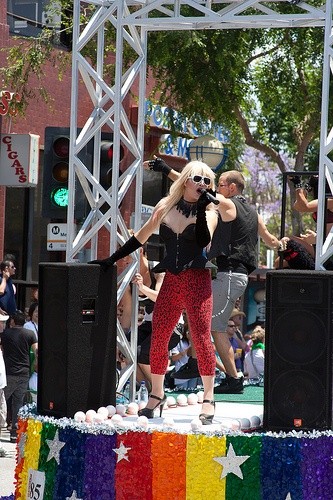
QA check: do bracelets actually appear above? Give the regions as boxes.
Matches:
[295,188,301,192]
[180,352,183,356]
[278,241,283,252]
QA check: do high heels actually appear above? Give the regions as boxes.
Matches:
[199,400,215,424]
[138,393,167,418]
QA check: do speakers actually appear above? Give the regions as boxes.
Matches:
[35,262,116,419]
[263,269,333,433]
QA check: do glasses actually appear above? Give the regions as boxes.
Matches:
[216,182,230,189]
[7,265,16,269]
[188,175,211,186]
[227,324,237,328]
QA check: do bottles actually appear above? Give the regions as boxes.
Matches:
[123,381,130,404]
[138,381,148,403]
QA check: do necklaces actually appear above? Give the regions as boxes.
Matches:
[176,194,207,219]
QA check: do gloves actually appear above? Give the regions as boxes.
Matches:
[87,234,142,271]
[193,188,217,247]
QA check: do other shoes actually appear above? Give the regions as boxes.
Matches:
[10,433,19,443]
[214,372,245,394]
[0,447,7,457]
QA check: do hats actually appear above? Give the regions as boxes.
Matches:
[229,307,247,319]
[0,313,9,321]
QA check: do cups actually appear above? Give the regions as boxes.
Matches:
[236,348,242,357]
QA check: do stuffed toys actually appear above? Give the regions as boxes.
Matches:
[274,239,315,270]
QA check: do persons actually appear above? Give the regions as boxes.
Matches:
[161,297,265,393]
[0,254,39,457]
[119,228,186,399]
[142,154,282,394]
[293,174,333,271]
[88,160,233,422]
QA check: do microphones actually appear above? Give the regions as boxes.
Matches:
[201,189,219,205]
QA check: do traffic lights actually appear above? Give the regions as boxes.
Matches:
[88,131,124,219]
[42,127,88,220]
